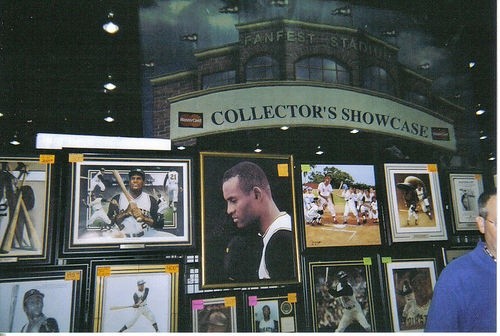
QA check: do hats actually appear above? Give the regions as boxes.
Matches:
[22,288,44,303]
[205,313,228,326]
[226,233,265,282]
[137,279,145,285]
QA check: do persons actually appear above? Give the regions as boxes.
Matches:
[0,159,478,333]
[424,187,497,333]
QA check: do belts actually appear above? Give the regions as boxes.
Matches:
[125,231,144,238]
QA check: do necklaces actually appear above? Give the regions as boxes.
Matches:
[262,212,287,237]
[484,245,497,263]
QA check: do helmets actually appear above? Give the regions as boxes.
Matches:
[338,271,348,279]
[128,168,145,180]
[395,175,427,196]
